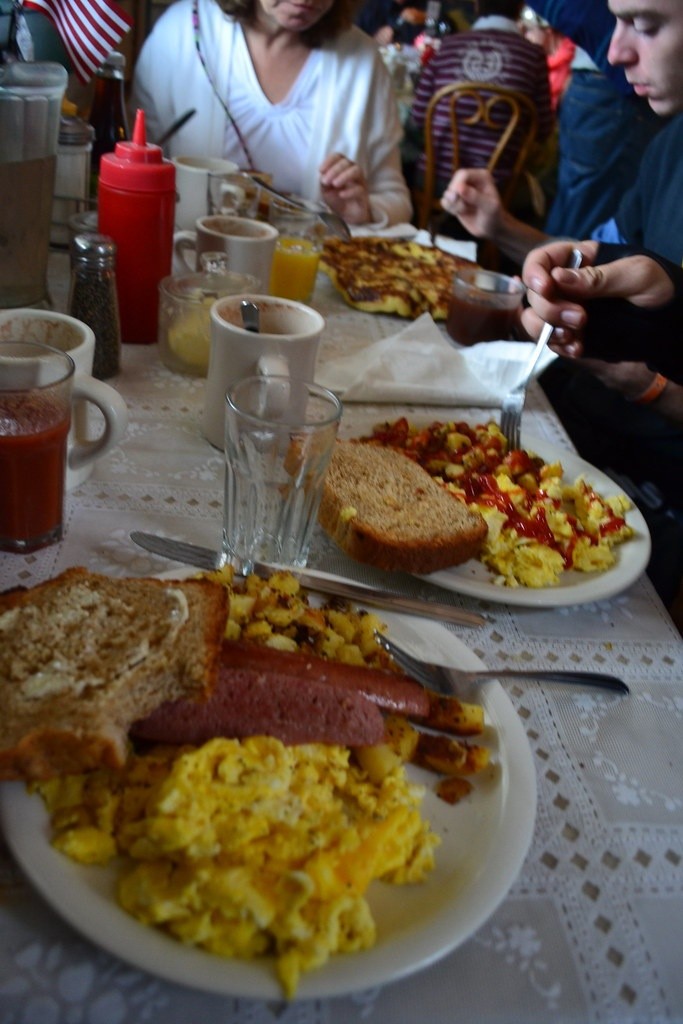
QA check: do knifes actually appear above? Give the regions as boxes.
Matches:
[131,531,486,629]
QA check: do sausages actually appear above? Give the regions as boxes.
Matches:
[116,639,433,747]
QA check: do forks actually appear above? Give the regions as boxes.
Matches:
[373,629,630,696]
[500,250,584,454]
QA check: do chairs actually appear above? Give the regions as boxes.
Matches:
[406,83,539,246]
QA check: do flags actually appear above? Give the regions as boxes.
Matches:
[20,0,136,86]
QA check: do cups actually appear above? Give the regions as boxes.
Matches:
[220,375,343,572]
[268,194,331,307]
[449,269,525,346]
[203,295,325,454]
[172,155,245,228]
[0,307,128,491]
[159,271,259,379]
[1,338,73,556]
[172,215,279,296]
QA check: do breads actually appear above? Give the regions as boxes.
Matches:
[0,565,231,785]
[282,440,487,577]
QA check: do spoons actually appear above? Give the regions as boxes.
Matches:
[242,172,353,243]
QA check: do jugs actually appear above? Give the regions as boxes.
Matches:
[0,62,69,321]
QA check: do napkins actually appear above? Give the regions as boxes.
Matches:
[308,311,559,404]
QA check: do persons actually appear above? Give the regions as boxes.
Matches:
[129,0,417,234]
[348,0,683,606]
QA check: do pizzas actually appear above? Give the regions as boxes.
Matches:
[311,235,489,320]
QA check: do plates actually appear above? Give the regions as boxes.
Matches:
[407,448,651,608]
[1,565,540,1002]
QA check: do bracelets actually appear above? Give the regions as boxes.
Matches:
[625,371,668,407]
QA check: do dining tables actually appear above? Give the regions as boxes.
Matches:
[0,268,683,1024]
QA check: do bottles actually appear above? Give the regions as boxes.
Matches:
[97,108,175,345]
[47,121,93,246]
[67,230,122,382]
